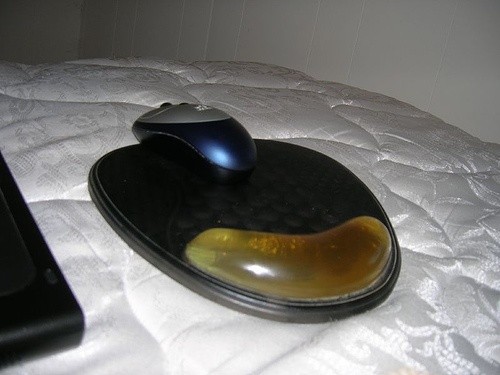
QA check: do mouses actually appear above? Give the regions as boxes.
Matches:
[131,102,258,180]
